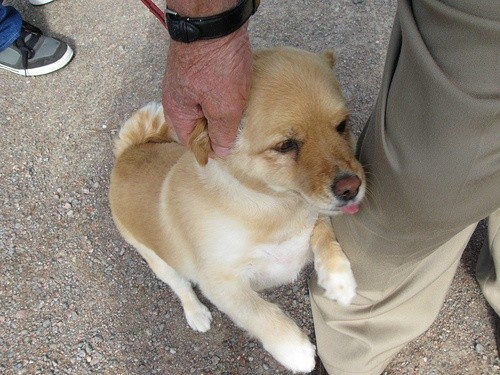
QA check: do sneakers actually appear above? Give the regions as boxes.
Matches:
[0,23,73,75]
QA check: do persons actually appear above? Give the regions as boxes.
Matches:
[0,0,73,77]
[162,0,499,375]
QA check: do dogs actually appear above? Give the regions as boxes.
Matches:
[108,44,369,375]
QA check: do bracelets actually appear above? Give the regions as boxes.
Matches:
[142,0,261,42]
